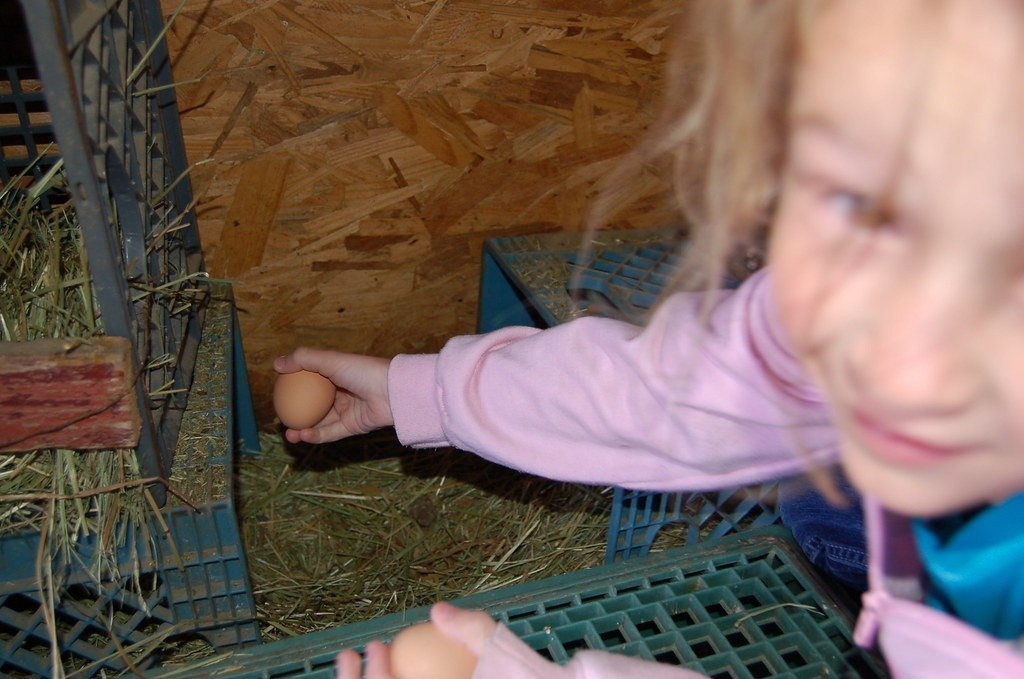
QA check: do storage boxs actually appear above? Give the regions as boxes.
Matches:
[0,0,214,517]
[121,526,892,678]
[473,227,789,565]
[0,281,263,679]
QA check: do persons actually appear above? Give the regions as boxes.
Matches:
[273,0,1024,679]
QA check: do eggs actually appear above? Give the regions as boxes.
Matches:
[389,622,476,679]
[273,370,336,430]
